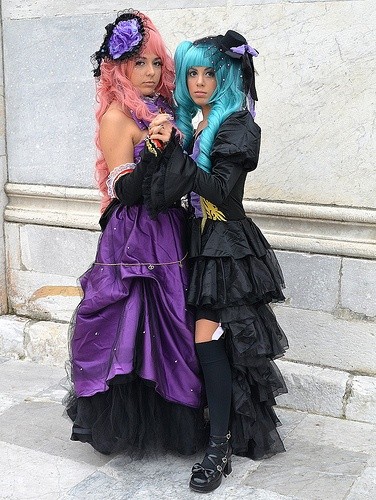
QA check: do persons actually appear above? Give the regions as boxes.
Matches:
[148,31,283,493]
[67,7,210,459]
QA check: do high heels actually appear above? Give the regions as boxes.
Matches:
[187,439,234,493]
